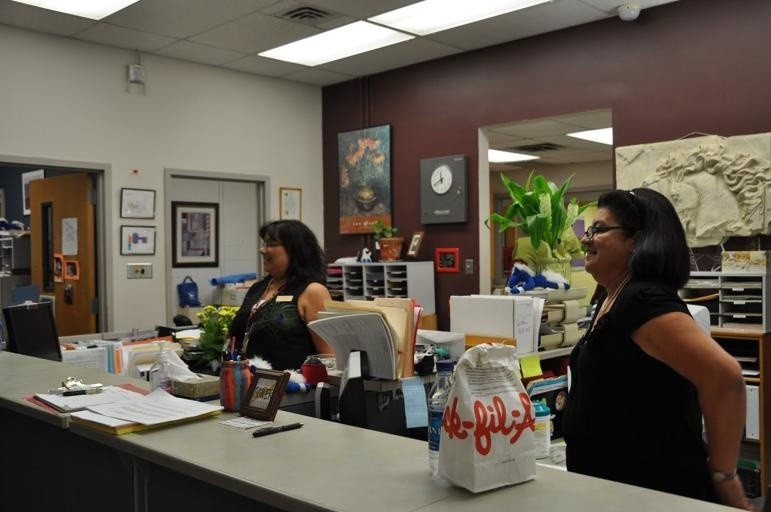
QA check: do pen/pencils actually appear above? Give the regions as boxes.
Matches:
[253,423,303,437]
[63,389,102,395]
[223,336,247,361]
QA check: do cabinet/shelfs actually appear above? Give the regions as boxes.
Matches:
[325,261,437,331]
[680,262,771,333]
[709,326,770,512]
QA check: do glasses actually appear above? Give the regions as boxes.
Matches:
[585,224,631,239]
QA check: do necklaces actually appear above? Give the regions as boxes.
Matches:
[599,272,630,311]
[255,278,286,315]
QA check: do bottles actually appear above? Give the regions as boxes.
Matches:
[427,360,457,479]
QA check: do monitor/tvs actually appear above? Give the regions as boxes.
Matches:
[3,301,62,361]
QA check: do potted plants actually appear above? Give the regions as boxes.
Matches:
[484,170,597,286]
[372,220,404,262]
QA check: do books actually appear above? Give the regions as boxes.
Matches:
[307,288,593,398]
[32,382,219,436]
[682,277,762,377]
[60,327,205,379]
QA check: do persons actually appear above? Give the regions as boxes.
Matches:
[232,219,333,370]
[561,188,756,511]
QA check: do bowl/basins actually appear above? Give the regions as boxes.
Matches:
[309,354,337,370]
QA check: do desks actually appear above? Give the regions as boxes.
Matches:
[0,349,216,512]
[73,395,749,511]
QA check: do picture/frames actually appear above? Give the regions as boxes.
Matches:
[336,125,393,234]
[120,187,156,219]
[407,231,425,257]
[120,225,156,256]
[433,248,460,273]
[170,201,219,268]
[280,188,302,223]
[127,263,153,280]
[238,369,291,423]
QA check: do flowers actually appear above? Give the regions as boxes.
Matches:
[194,303,242,365]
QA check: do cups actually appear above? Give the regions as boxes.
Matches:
[219,360,253,410]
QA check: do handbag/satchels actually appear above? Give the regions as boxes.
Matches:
[433,333,539,497]
[178,276,201,307]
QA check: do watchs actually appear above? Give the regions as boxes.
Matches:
[709,469,735,482]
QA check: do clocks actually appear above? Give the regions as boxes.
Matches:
[419,155,467,224]
